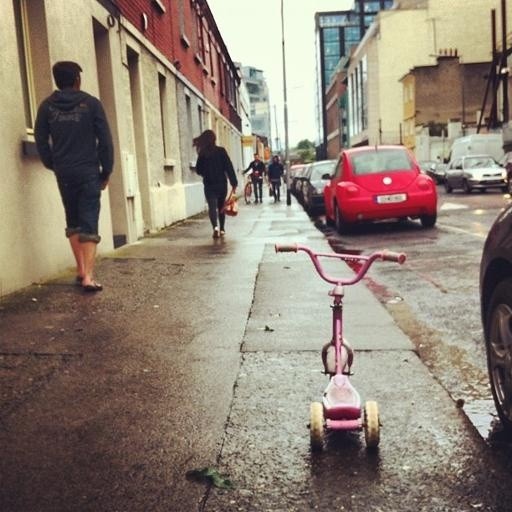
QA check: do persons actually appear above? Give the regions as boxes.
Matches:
[268,155,286,204]
[189,130,237,236]
[240,153,268,203]
[33,62,115,291]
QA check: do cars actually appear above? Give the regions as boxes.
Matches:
[426,164,448,184]
[480,202,512,432]
[290,145,437,235]
[443,155,509,194]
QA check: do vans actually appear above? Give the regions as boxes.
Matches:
[446,135,510,167]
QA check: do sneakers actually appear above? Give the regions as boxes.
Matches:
[75,275,104,292]
[211,226,225,238]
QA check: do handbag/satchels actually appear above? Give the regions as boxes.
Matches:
[219,188,240,217]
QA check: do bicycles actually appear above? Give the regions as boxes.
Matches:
[242,172,258,205]
